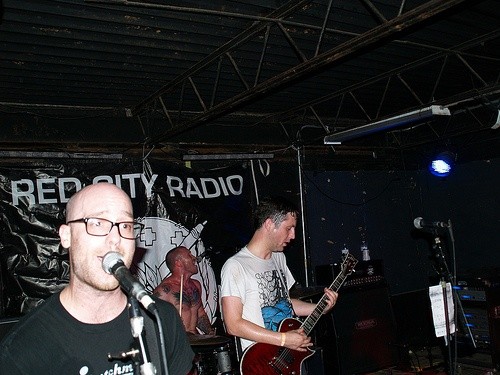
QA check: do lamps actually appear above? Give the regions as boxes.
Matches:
[431,147,458,174]
[324,105,451,145]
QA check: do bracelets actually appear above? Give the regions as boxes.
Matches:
[281,332,286,347]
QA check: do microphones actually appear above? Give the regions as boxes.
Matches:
[102,251,157,314]
[414,217,445,229]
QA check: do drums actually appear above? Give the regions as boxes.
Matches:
[188,336,239,375]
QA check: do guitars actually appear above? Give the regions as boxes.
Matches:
[240,254,359,375]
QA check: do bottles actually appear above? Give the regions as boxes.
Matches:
[360,240,368,255]
[341,244,350,254]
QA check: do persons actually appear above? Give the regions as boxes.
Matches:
[220,198,338,352]
[0,181,196,375]
[153,246,214,336]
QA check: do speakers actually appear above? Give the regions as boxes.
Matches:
[295,277,401,375]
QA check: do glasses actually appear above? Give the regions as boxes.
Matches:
[67,218,145,240]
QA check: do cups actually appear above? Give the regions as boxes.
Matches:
[363,250,371,260]
